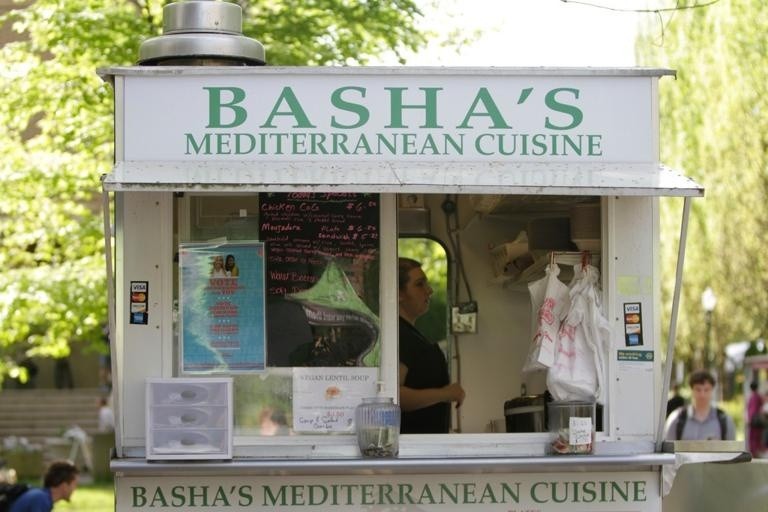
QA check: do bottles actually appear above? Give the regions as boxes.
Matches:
[354,396,403,460]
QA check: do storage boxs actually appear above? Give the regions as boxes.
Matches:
[145,379,234,459]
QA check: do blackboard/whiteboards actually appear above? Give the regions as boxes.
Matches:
[258,192,381,368]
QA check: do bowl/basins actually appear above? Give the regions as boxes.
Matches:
[571,237,602,252]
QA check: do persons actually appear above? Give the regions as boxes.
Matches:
[666,383,685,415]
[95,395,115,433]
[745,380,768,458]
[208,254,227,278]
[224,254,240,277]
[0,461,79,512]
[398,256,465,433]
[662,367,738,440]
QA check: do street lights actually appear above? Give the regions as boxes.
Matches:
[701,286,716,369]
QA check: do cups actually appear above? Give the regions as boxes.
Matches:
[550,400,598,455]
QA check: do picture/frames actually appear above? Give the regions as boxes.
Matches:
[178,240,268,375]
